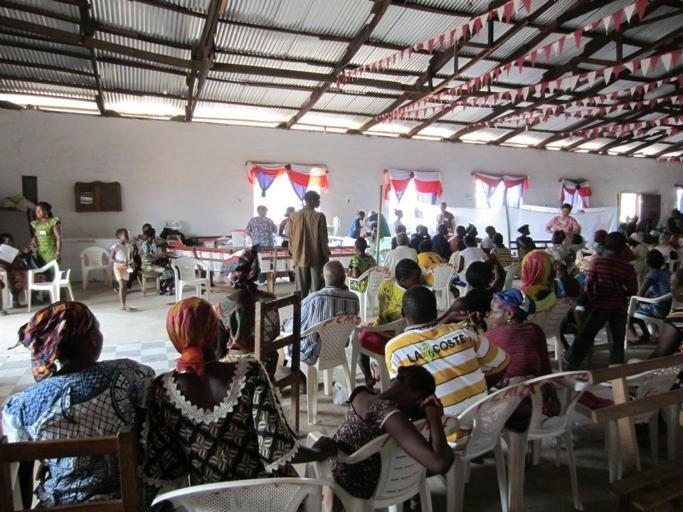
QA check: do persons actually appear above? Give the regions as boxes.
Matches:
[2,190,682,511]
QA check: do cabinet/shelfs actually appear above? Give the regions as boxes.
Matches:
[0,207,34,248]
[640,193,660,223]
[74,180,123,212]
